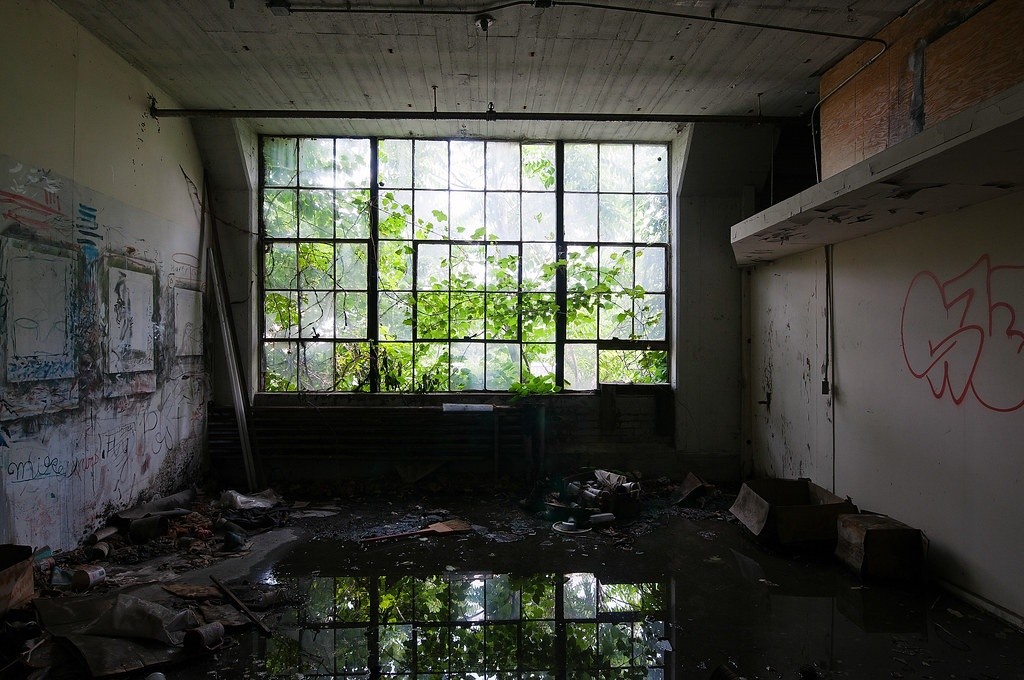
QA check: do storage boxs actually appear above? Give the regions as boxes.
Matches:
[835,513,925,578]
[0,544,37,614]
[732,477,851,543]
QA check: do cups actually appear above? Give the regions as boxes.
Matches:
[73,566,105,593]
[186,622,225,651]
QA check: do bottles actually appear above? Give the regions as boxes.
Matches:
[560,479,615,530]
[220,518,249,552]
